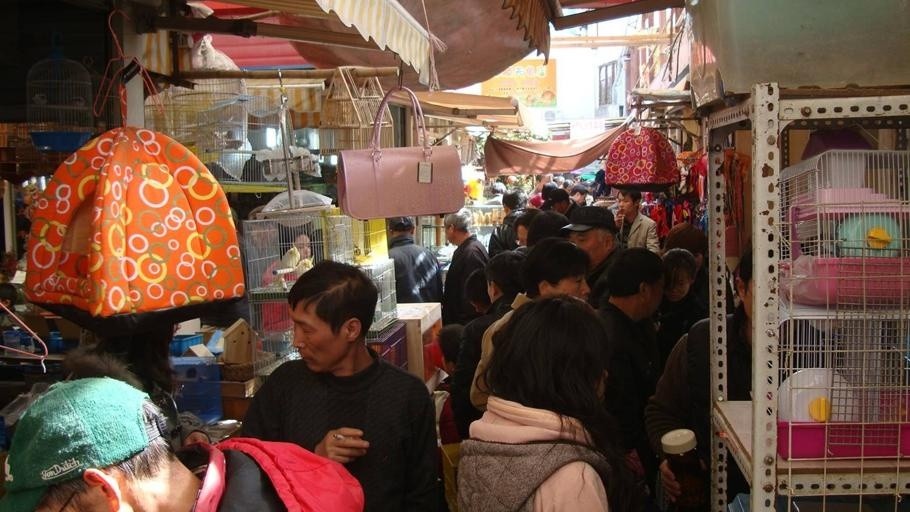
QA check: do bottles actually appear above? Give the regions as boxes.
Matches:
[660,426,707,509]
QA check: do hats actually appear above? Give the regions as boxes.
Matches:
[546,189,569,206]
[560,206,614,234]
[0,375,168,512]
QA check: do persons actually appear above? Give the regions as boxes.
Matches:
[387,215,443,304]
[260,232,316,333]
[1,376,366,512]
[236,259,442,512]
[76,359,212,447]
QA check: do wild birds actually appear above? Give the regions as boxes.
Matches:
[280,246,301,268]
[296,259,313,279]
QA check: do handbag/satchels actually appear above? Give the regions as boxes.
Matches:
[331,88,465,218]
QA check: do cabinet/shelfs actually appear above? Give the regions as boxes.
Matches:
[701,72,907,510]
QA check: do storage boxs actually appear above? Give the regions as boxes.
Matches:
[397,301,446,393]
[365,319,409,373]
